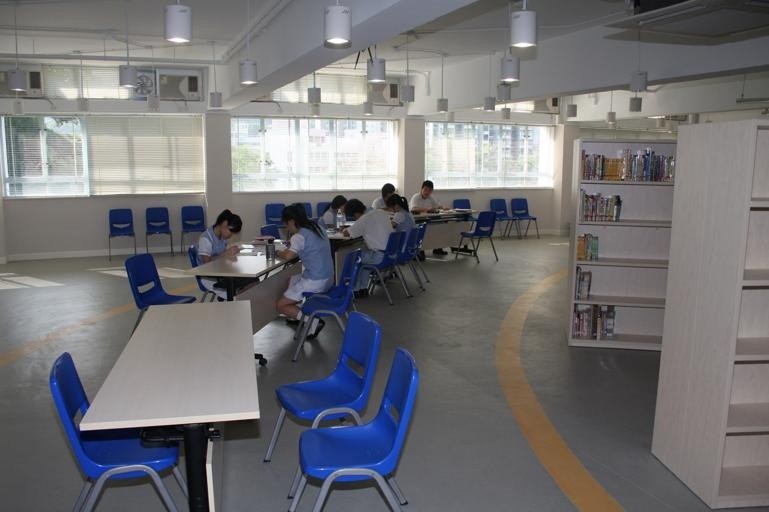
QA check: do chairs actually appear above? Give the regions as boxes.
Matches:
[354,231,412,306]
[453,199,478,231]
[146,207,174,256]
[260,224,280,240]
[264,310,381,498]
[289,345,419,511]
[301,247,362,319]
[490,198,519,240]
[370,227,426,295]
[49,352,188,512]
[180,206,205,256]
[108,208,137,261]
[188,244,240,303]
[265,203,290,239]
[317,202,331,219]
[455,211,498,264]
[510,198,540,239]
[304,202,312,221]
[125,253,197,339]
[388,222,430,291]
[293,256,363,362]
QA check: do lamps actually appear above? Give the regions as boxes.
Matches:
[436,57,448,112]
[164,0,191,43]
[147,48,161,112]
[566,95,576,117]
[401,35,414,102]
[608,91,616,125]
[239,1,258,85]
[367,44,385,82]
[119,23,138,88]
[501,49,520,82]
[210,43,222,106]
[632,29,648,92]
[309,102,320,117]
[363,100,373,115]
[510,0,536,49]
[323,0,352,49]
[496,82,511,101]
[77,58,89,112]
[502,104,510,119]
[12,92,23,115]
[484,55,495,111]
[308,72,321,103]
[630,92,643,111]
[6,7,27,92]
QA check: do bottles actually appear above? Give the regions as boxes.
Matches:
[265,237,277,260]
[336,210,344,230]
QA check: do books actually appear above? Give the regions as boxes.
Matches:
[421,207,473,216]
[571,304,616,339]
[326,224,352,234]
[577,234,599,263]
[576,267,593,300]
[229,236,289,256]
[582,190,623,222]
[582,147,674,182]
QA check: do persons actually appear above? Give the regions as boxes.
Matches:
[196,210,242,302]
[371,182,396,210]
[343,198,390,300]
[275,201,335,341]
[386,194,416,279]
[409,180,451,262]
[321,196,348,228]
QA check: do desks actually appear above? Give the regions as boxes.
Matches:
[79,300,260,512]
[414,209,481,257]
[188,238,299,367]
[325,221,364,290]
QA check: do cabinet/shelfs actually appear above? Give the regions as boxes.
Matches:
[651,119,768,508]
[567,137,678,351]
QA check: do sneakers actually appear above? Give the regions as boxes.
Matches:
[286,317,300,326]
[354,290,361,299]
[419,251,425,262]
[307,318,325,340]
[433,248,448,255]
[362,289,369,297]
